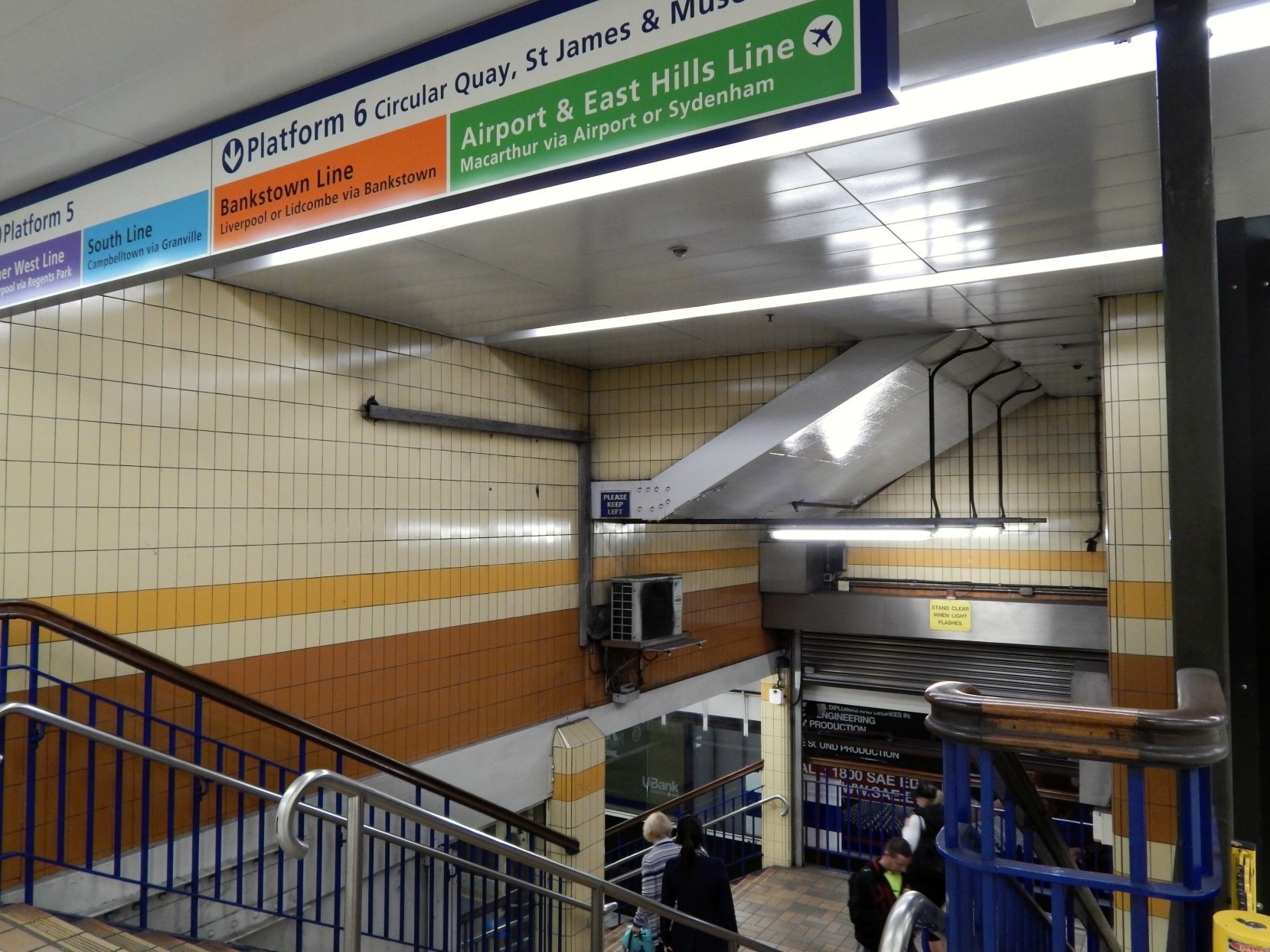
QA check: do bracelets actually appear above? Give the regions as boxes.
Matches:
[633,922,642,928]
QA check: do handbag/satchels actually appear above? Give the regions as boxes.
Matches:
[622,925,655,952]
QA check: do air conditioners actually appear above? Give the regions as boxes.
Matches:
[610,572,684,643]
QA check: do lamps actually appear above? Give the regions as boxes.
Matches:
[767,521,1006,541]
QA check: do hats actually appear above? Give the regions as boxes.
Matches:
[910,783,936,799]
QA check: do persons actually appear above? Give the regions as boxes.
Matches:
[632,812,741,952]
[849,784,946,952]
